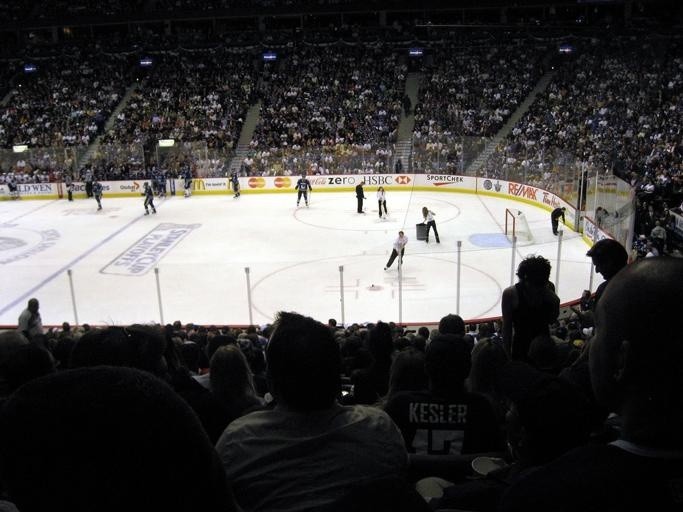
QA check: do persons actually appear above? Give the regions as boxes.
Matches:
[500,256,561,363]
[527,0,683,512]
[1,298,529,512]
[1,1,527,215]
[384,231,408,271]
[421,207,441,245]
